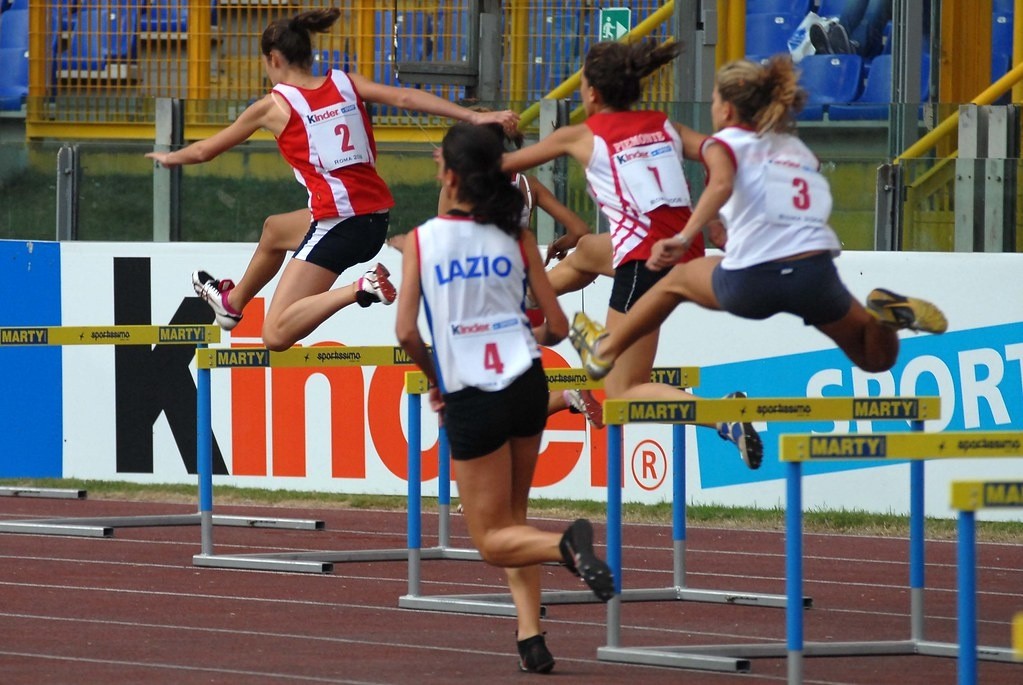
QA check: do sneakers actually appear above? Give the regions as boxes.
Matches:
[569,311,615,381]
[516,630,555,673]
[866,287,948,335]
[192,269,244,330]
[568,390,606,429]
[356,263,397,308]
[560,519,615,600]
[717,392,764,470]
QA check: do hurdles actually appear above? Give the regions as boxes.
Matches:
[594,395,1023,674]
[0,324,326,539]
[776,430,1023,685]
[395,364,813,619]
[947,479,1023,685]
[190,345,565,574]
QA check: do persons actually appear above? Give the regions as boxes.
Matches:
[395,121,615,673]
[386,106,604,430]
[433,41,763,469]
[568,55,948,374]
[144,7,522,352]
[809,0,932,58]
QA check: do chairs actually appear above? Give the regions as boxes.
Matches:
[245,0,675,125]
[0,0,224,118]
[745,0,1013,120]
